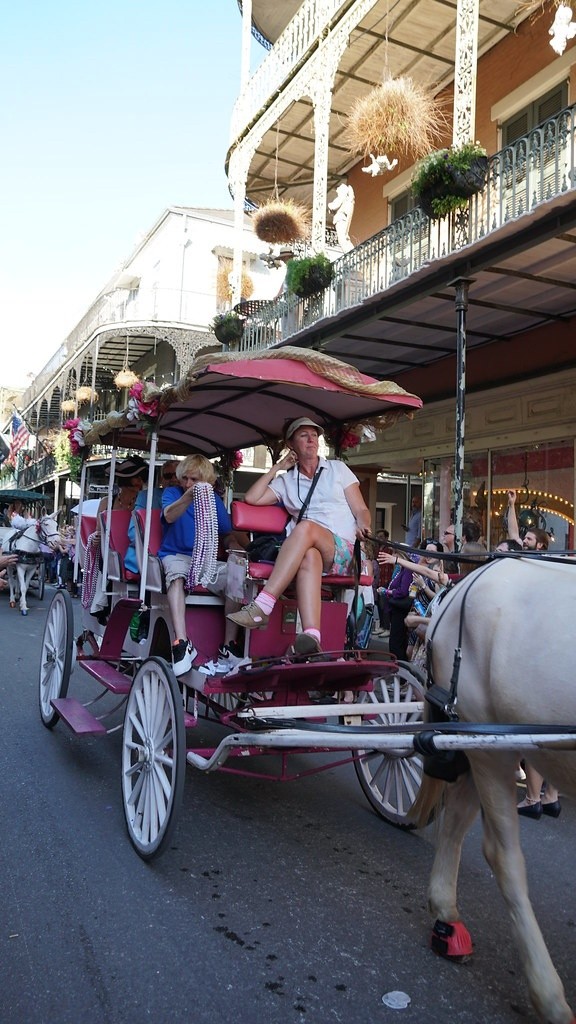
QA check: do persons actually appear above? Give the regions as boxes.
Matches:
[91,452,150,612]
[1,503,81,598]
[225,417,372,660]
[123,460,183,643]
[43,439,54,457]
[274,246,299,340]
[158,455,252,676]
[328,183,360,271]
[361,491,560,818]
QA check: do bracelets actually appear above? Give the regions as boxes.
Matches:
[395,556,398,564]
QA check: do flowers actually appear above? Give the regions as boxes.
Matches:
[325,428,360,459]
[228,449,245,470]
[124,381,159,438]
[207,313,245,344]
[63,419,94,453]
[412,140,489,219]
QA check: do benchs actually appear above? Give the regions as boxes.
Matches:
[87,496,375,657]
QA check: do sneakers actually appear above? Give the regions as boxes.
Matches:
[217,640,247,667]
[171,636,198,678]
[225,600,269,631]
[292,631,324,661]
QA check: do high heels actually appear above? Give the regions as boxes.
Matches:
[539,800,562,818]
[514,801,543,820]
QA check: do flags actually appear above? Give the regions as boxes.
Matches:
[0,409,30,463]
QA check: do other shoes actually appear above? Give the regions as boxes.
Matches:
[514,777,529,788]
[51,580,66,589]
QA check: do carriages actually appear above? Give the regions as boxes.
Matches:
[38,344,575,1023]
[0,490,62,613]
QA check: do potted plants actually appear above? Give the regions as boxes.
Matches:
[285,254,332,297]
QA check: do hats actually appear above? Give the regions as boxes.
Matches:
[101,454,150,483]
[284,416,325,445]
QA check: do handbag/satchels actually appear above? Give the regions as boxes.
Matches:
[247,535,282,564]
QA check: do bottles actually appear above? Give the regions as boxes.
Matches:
[413,599,426,616]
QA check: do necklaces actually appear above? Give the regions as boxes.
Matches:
[186,482,218,588]
[81,532,100,609]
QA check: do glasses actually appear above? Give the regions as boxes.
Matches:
[162,472,178,481]
[425,539,438,545]
[443,530,455,536]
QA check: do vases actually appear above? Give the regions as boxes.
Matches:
[421,156,486,218]
[213,319,243,342]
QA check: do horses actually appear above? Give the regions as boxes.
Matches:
[406,555,576,1024]
[1,506,61,610]
